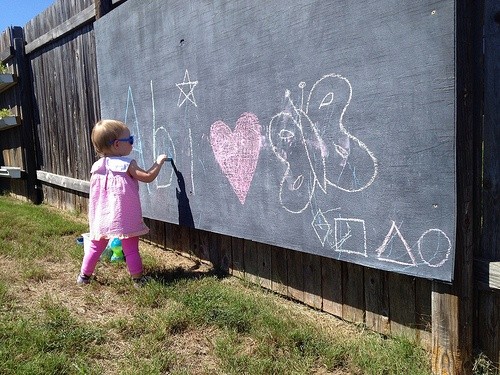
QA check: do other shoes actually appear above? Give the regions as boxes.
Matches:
[132,275,153,288]
[77,272,96,283]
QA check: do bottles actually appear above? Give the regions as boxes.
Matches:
[110,238,124,263]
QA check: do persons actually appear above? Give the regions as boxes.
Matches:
[77,119,168,286]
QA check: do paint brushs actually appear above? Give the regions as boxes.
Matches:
[161,157,172,161]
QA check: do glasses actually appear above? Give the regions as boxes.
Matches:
[108,135,134,146]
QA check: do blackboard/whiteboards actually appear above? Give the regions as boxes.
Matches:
[92,0,457,282]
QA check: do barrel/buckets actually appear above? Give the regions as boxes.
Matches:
[81,232,107,261]
